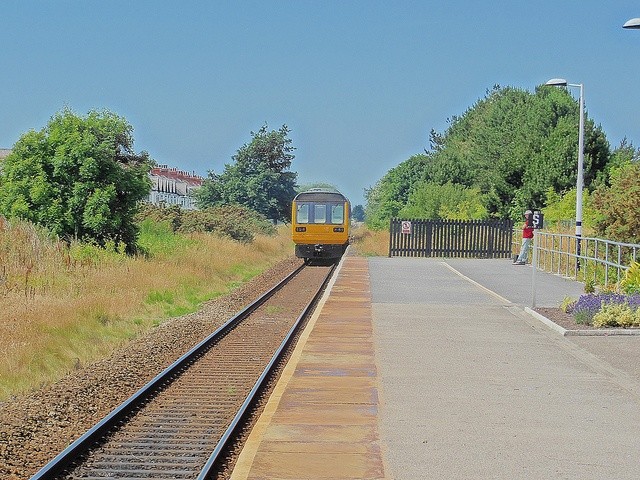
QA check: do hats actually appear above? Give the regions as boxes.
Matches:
[524,210,532,214]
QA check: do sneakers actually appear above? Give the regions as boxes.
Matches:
[521,262,525,265]
[512,261,521,265]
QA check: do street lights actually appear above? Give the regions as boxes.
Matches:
[624,18,640,28]
[545,79,584,270]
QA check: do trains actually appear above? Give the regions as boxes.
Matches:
[292,187,351,263]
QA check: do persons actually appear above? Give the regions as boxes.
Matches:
[512,210,534,265]
